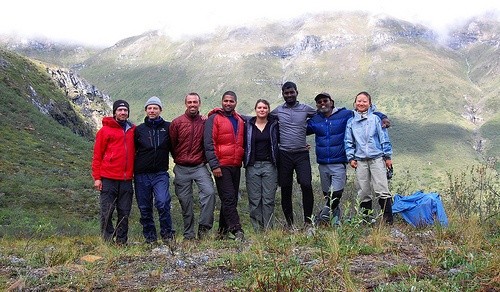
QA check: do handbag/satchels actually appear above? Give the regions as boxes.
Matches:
[392,191,448,228]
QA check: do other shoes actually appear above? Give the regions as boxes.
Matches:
[234,231,245,241]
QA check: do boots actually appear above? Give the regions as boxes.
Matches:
[359,199,372,226]
[378,197,394,227]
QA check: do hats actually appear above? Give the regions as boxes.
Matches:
[314,92,331,101]
[113,99,130,117]
[144,96,162,111]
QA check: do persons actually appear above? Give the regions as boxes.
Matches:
[306,93,391,224]
[92,100,137,245]
[134,96,176,246]
[204,91,245,240]
[344,91,394,225]
[238,82,316,231]
[244,99,311,231]
[168,93,216,242]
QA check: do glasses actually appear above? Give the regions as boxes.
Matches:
[317,99,328,104]
